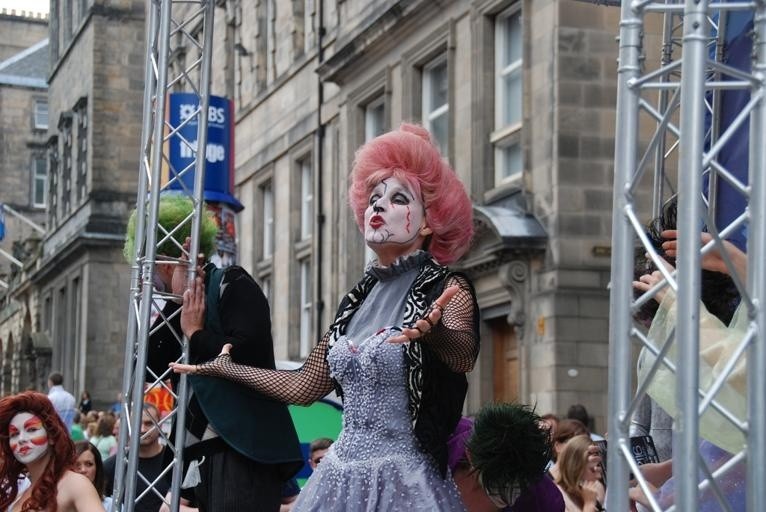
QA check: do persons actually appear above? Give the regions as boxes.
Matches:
[1,370,166,512]
[628,391,675,509]
[631,227,750,460]
[165,121,482,512]
[449,402,567,510]
[628,196,742,344]
[280,438,335,512]
[538,405,608,510]
[123,193,305,512]
